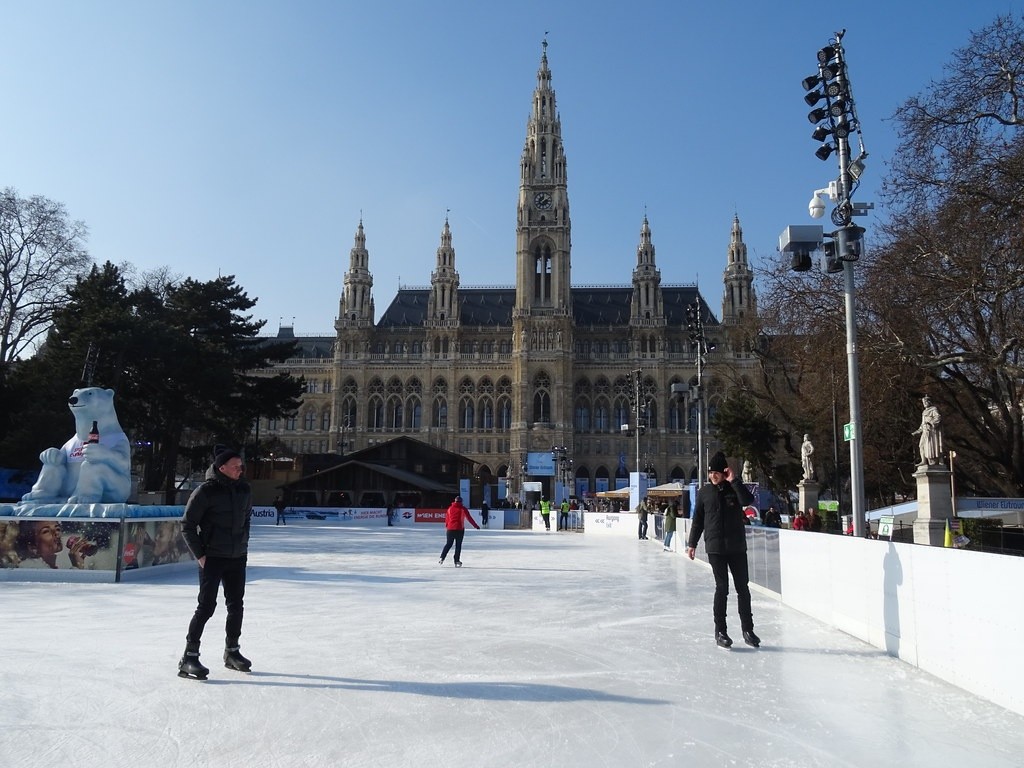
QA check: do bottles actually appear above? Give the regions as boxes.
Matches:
[89,421,99,445]
[54,534,98,556]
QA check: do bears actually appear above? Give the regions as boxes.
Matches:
[20,385,132,504]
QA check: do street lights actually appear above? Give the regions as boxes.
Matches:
[671,294,709,491]
[620,367,646,472]
[551,445,571,486]
[779,26,875,539]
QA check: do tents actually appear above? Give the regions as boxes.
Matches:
[647,480,688,496]
[597,486,630,498]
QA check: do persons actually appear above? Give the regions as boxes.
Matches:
[539,496,551,528]
[437,495,480,568]
[793,510,811,531]
[741,460,752,482]
[0,521,88,569]
[688,450,761,648]
[663,500,680,552]
[481,500,492,529]
[180,444,252,680]
[911,396,941,467]
[124,522,180,571]
[847,517,853,536]
[386,504,394,526]
[273,495,287,525]
[638,497,664,540]
[764,507,783,529]
[559,496,686,530]
[805,507,823,533]
[494,498,527,510]
[800,434,815,481]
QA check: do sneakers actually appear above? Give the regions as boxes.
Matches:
[715,630,733,650]
[177,648,209,680]
[743,628,760,648]
[223,646,252,673]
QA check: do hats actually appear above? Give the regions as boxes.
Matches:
[455,496,463,502]
[214,444,242,470]
[708,452,728,475]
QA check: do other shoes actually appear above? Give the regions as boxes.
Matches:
[439,557,443,564]
[664,546,673,552]
[454,561,462,568]
[639,536,648,540]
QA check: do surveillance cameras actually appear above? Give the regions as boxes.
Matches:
[809,197,825,219]
